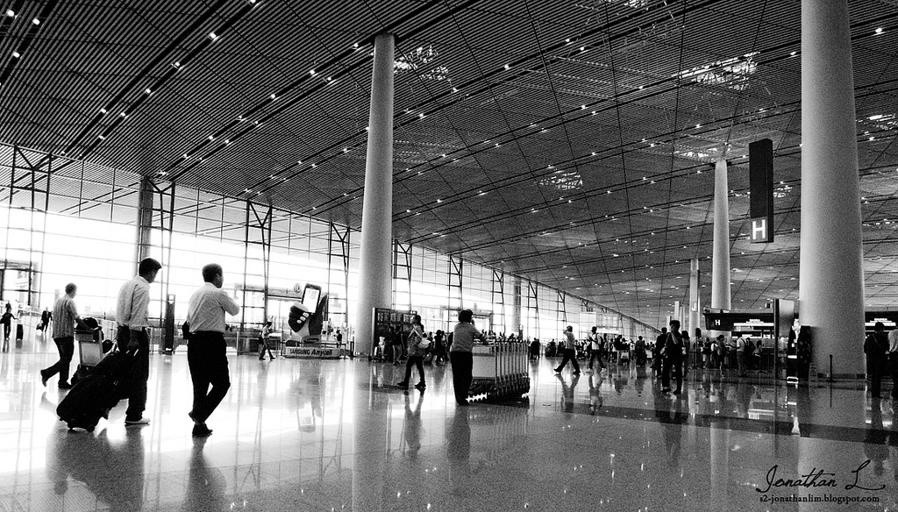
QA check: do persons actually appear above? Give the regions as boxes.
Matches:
[0,300,897,413]
[182,262,239,434]
[38,283,89,388]
[116,258,162,423]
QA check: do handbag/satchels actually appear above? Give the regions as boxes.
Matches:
[417,337,432,350]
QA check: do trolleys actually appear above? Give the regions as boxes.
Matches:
[465,338,529,400]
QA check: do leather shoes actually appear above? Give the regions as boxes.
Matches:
[59,383,73,389]
[415,381,426,387]
[189,409,213,436]
[397,381,409,387]
[125,417,151,423]
[41,369,51,387]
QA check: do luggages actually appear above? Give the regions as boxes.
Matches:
[57,345,143,432]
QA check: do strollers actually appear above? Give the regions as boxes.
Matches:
[71,326,120,387]
